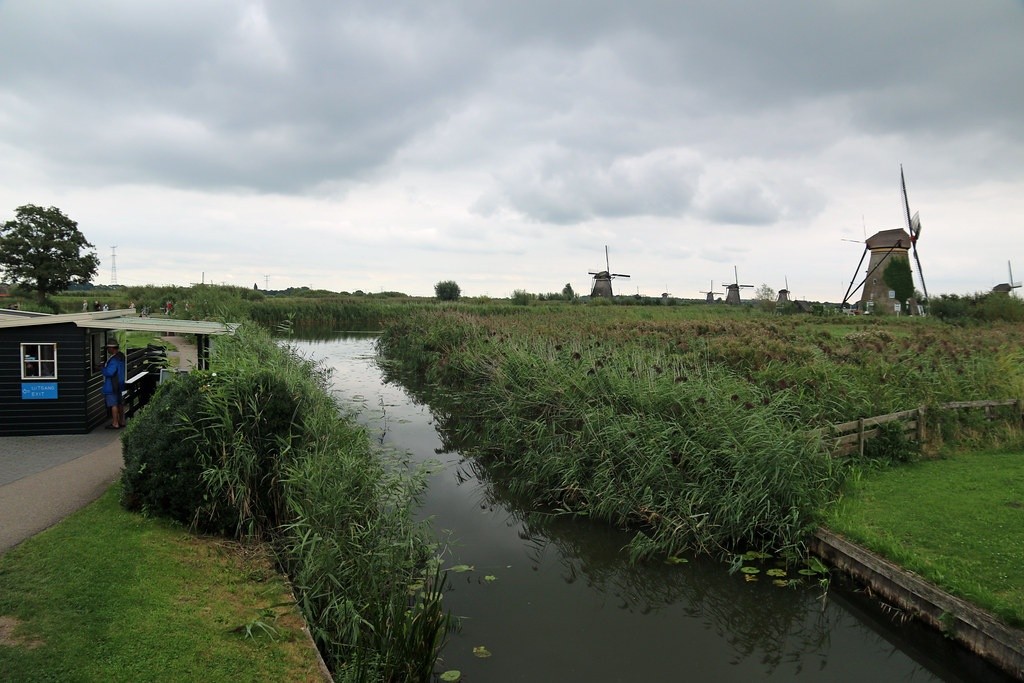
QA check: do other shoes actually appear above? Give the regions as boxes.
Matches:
[104,422,125,428]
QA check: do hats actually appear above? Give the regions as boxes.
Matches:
[107,337,118,347]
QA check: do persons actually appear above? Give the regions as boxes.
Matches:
[96,337,127,430]
[129,298,210,318]
[82,299,109,312]
